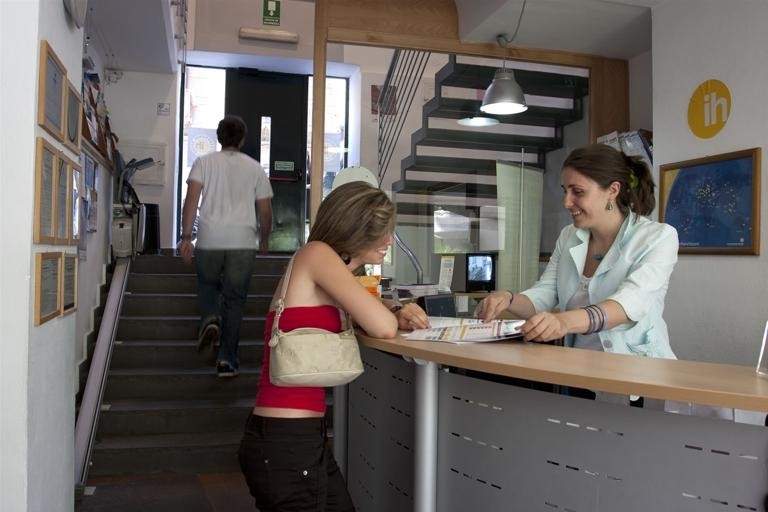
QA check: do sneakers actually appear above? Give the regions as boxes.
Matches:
[216,358,243,378]
[195,314,221,362]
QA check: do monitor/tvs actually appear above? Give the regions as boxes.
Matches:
[417,294,456,317]
[431,252,496,292]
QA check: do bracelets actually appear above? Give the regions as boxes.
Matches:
[179,233,192,241]
[579,303,608,336]
[388,305,402,314]
[503,289,516,305]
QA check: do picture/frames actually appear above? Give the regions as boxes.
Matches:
[656,146,765,259]
[32,37,86,328]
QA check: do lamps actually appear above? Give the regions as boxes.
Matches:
[478,0,532,117]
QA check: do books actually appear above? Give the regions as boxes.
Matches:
[400,314,526,345]
[390,281,439,298]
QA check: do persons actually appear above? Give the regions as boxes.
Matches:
[237,180,431,511]
[176,113,275,378]
[471,141,680,409]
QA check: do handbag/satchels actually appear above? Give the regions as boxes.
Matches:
[267,317,366,388]
